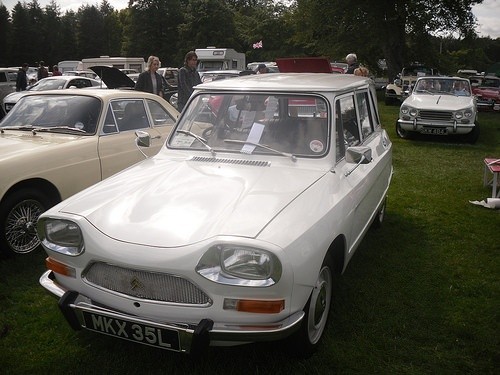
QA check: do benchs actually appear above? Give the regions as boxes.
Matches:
[256,117,364,147]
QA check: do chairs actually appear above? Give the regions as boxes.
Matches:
[109,103,149,132]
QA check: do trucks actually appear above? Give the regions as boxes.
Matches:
[194,46,246,75]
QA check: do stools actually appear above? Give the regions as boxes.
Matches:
[483,158,500,198]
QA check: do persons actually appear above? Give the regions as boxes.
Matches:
[16,61,62,94]
[135,56,165,97]
[345,54,359,75]
[450,81,471,96]
[177,51,202,113]
[258,64,275,73]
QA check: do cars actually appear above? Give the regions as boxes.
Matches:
[395,75,481,144]
[0,55,181,116]
[168,56,375,120]
[383,61,438,104]
[0,88,214,256]
[35,71,395,358]
[465,75,500,112]
